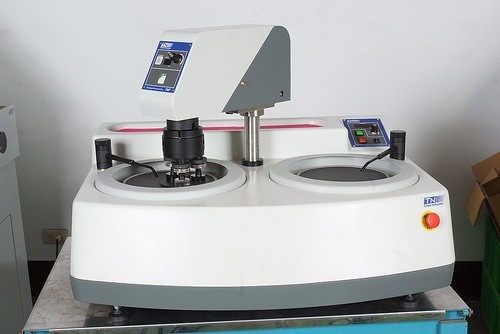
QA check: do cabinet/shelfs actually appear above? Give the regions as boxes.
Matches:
[1,104,33,334]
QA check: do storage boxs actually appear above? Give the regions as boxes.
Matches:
[465,151,500,239]
[480,262,500,334]
[483,213,500,301]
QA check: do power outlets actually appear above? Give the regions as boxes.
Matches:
[42,229,67,244]
